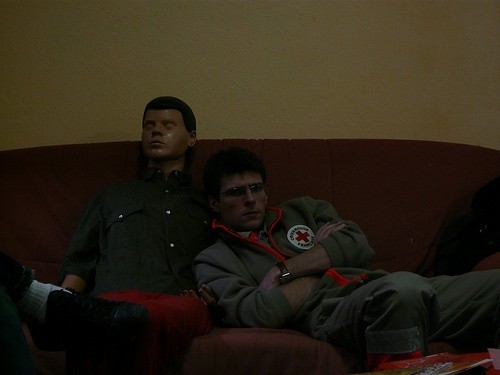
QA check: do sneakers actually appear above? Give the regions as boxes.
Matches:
[25,285,152,351]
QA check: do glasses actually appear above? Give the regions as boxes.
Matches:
[220,183,269,197]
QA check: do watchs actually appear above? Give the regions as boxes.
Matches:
[274,262,294,285]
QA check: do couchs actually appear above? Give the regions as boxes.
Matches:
[0,137,500,374]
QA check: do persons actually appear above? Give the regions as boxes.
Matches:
[64,96,220,310]
[191,143,500,375]
[0,253,144,374]
